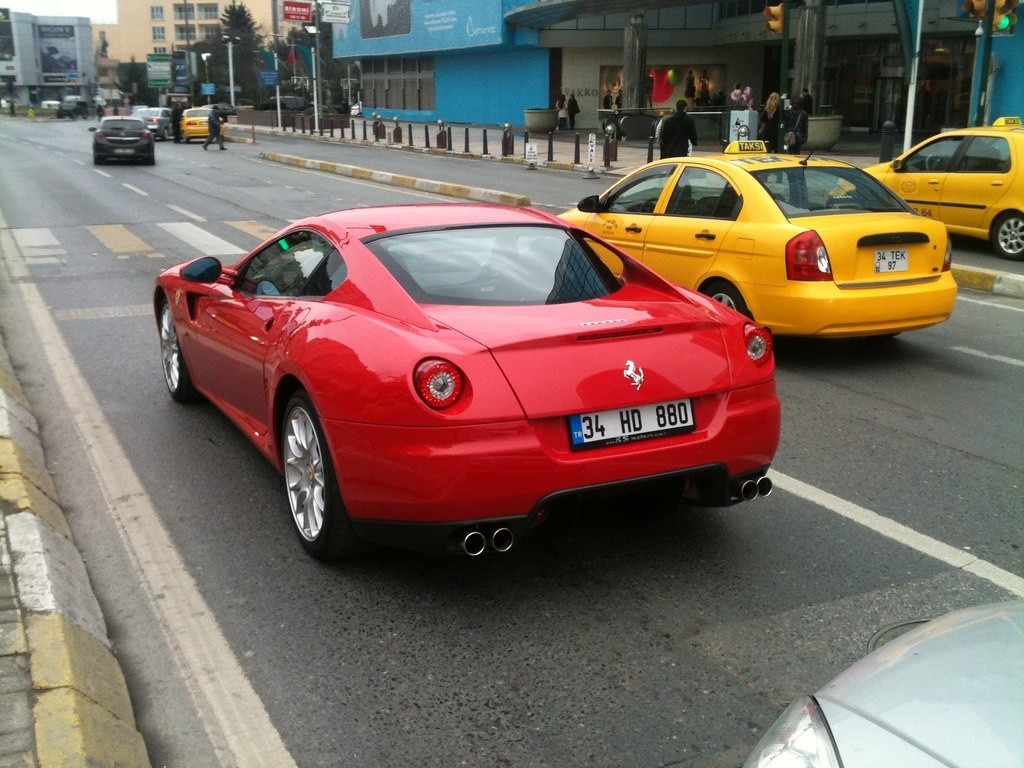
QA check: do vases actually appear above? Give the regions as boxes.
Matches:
[524,109,561,135]
[801,114,844,154]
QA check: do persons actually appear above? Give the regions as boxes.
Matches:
[96,105,104,122]
[112,106,119,115]
[171,103,182,143]
[183,102,191,109]
[8,102,16,117]
[759,88,812,183]
[203,106,228,150]
[694,90,726,106]
[615,89,622,107]
[342,97,349,112]
[655,100,698,158]
[730,84,752,102]
[556,95,568,130]
[568,95,577,129]
[685,70,709,107]
[603,90,613,109]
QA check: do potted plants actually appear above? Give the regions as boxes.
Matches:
[236,100,300,128]
[294,105,350,130]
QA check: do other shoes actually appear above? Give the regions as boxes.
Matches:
[220,147,228,150]
[202,145,207,149]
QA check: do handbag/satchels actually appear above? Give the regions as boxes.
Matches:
[784,132,796,146]
[758,123,765,136]
[576,105,580,113]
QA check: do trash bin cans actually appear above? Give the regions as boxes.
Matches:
[729,110,759,144]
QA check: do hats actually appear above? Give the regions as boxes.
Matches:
[213,106,219,109]
[676,100,687,109]
